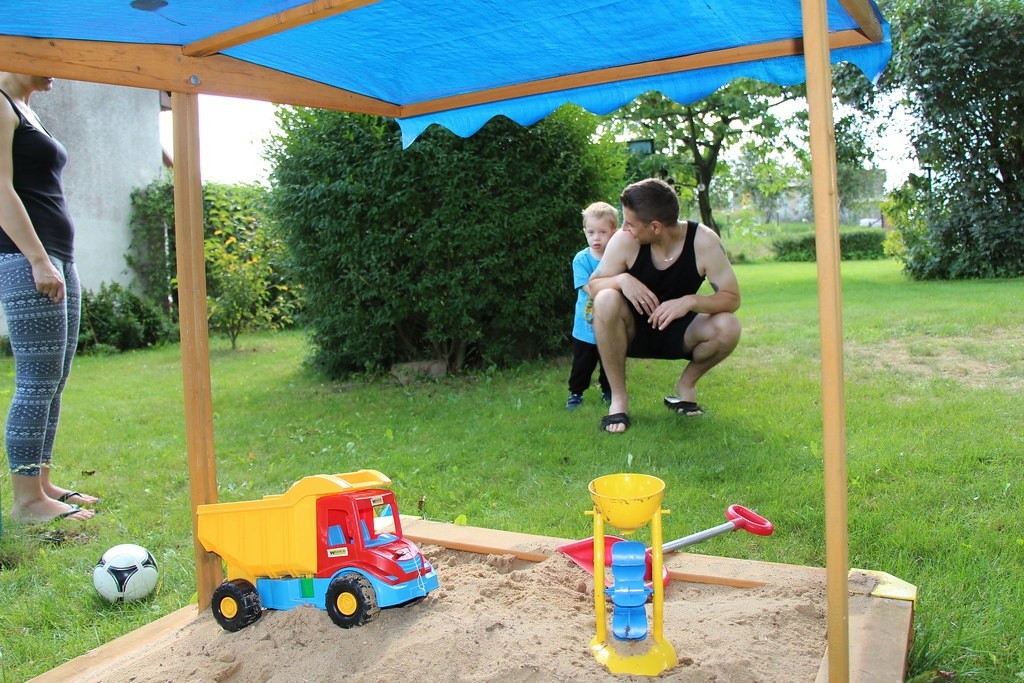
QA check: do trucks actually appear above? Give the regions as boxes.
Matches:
[194,470,440,634]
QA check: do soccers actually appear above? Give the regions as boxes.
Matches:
[92,542,159,602]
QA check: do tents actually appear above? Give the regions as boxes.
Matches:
[0,0,898,683]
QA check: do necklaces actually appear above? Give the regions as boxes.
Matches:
[652,223,682,262]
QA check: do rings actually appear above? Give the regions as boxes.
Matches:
[640,301,645,304]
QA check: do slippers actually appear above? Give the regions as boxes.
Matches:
[663,395,705,417]
[600,412,631,435]
[29,503,95,527]
[56,491,99,507]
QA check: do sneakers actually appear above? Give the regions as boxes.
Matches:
[601,392,611,405]
[565,394,584,410]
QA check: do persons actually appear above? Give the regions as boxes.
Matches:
[0,71,100,521]
[566,202,619,409]
[589,178,741,433]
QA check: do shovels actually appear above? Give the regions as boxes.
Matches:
[557,504,773,595]
